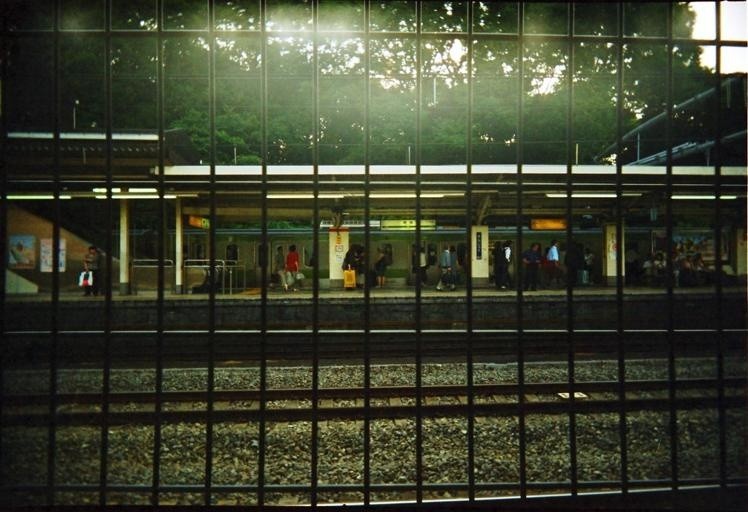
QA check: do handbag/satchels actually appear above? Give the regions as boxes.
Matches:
[78,270,93,287]
[441,271,457,284]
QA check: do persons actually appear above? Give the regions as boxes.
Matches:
[342,244,387,291]
[82,246,100,295]
[275,244,299,292]
[415,247,457,290]
[643,243,705,283]
[493,240,594,293]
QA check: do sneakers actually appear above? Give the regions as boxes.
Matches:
[436,288,441,291]
[451,287,456,291]
